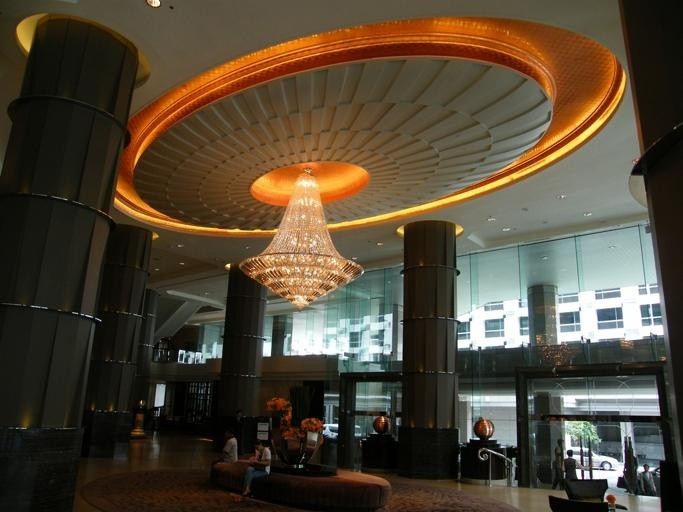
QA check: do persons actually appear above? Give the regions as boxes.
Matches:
[218,427,238,462]
[622,447,637,491]
[639,463,657,496]
[243,440,271,497]
[563,449,590,481]
[551,437,562,488]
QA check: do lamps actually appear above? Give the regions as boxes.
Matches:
[238,168,364,309]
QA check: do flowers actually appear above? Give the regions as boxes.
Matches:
[264,396,325,449]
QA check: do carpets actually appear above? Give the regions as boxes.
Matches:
[80,469,520,512]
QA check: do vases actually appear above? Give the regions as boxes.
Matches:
[269,436,319,469]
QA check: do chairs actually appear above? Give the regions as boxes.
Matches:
[547,479,626,512]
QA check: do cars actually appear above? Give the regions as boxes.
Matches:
[564,446,619,472]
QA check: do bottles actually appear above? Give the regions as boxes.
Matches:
[607,495,616,512]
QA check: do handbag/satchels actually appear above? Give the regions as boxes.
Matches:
[255,463,266,472]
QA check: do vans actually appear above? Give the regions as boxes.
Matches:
[322,423,361,438]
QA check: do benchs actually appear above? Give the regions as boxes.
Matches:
[211,460,391,512]
